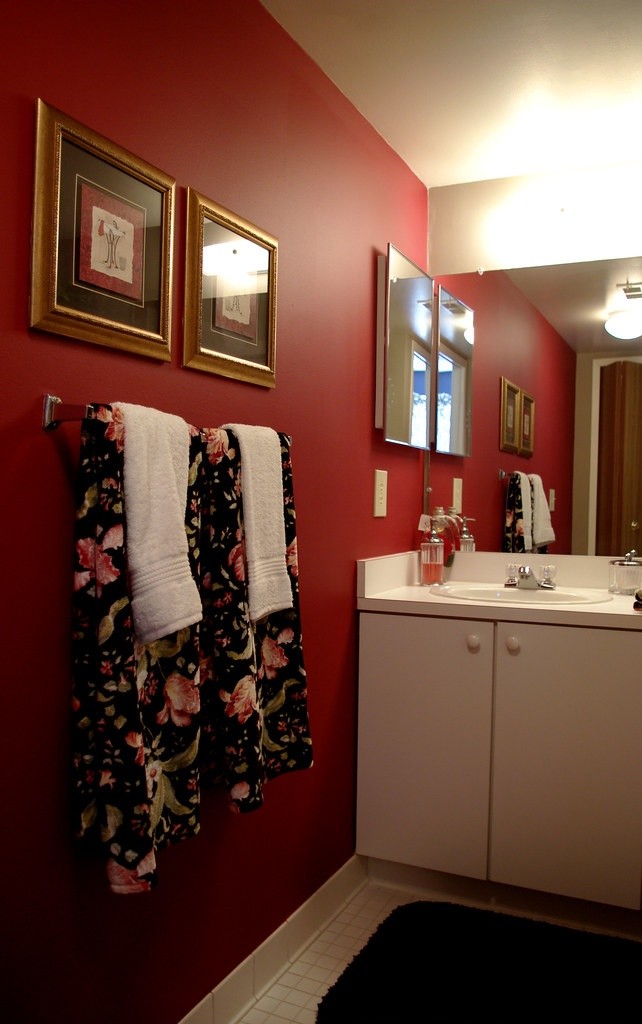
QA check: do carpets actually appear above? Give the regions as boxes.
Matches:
[315,900,642,1024]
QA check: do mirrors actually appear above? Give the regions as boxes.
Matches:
[433,256,642,556]
[435,283,476,458]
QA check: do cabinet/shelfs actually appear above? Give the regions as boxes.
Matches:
[354,599,642,910]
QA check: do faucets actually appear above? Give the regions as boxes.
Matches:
[502,564,558,590]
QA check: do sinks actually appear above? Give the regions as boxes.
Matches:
[429,580,614,603]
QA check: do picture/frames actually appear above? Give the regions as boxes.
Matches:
[518,390,535,457]
[384,241,433,452]
[183,185,280,389]
[499,375,522,453]
[29,97,178,362]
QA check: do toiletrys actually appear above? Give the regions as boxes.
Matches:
[420,518,445,586]
[458,517,476,552]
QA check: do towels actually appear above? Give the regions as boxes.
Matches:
[70,403,311,896]
[500,470,555,554]
